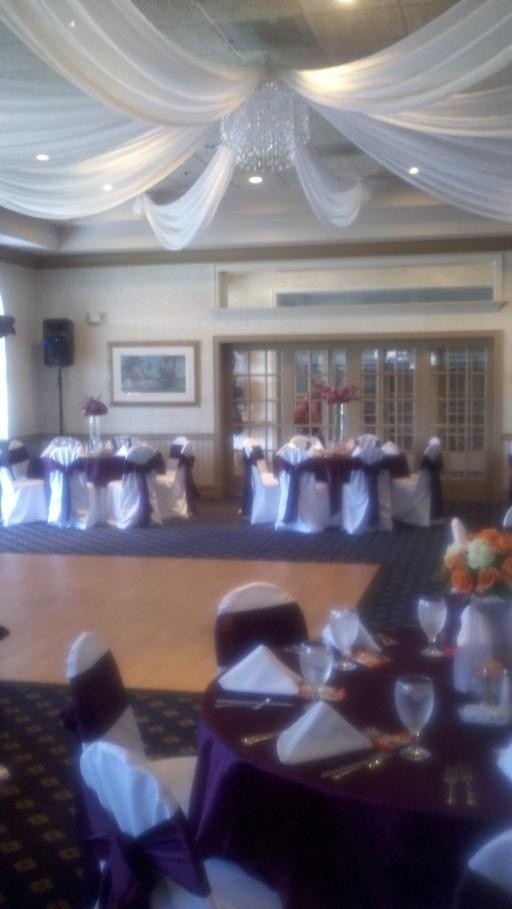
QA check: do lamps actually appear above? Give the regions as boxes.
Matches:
[220,80,311,175]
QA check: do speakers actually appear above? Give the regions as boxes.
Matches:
[42,318,75,366]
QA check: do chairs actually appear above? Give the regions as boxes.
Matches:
[79,742,282,909]
[341,445,392,534]
[41,447,103,530]
[0,449,45,528]
[449,831,512,908]
[391,436,444,528]
[276,446,343,533]
[213,583,311,672]
[121,444,161,529]
[289,435,324,452]
[8,440,42,482]
[355,434,381,450]
[66,629,200,819]
[41,435,83,459]
[242,439,280,525]
[156,437,190,523]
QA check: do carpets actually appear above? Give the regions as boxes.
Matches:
[1,499,512,906]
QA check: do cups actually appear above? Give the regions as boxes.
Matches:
[416,595,448,658]
[298,638,334,704]
[392,674,436,763]
[329,604,361,671]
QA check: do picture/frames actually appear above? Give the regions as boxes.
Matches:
[109,340,201,408]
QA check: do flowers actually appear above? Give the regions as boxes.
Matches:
[443,515,512,599]
[313,382,361,405]
[80,399,108,417]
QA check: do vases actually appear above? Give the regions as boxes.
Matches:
[328,402,341,445]
[455,601,511,695]
[89,418,102,449]
